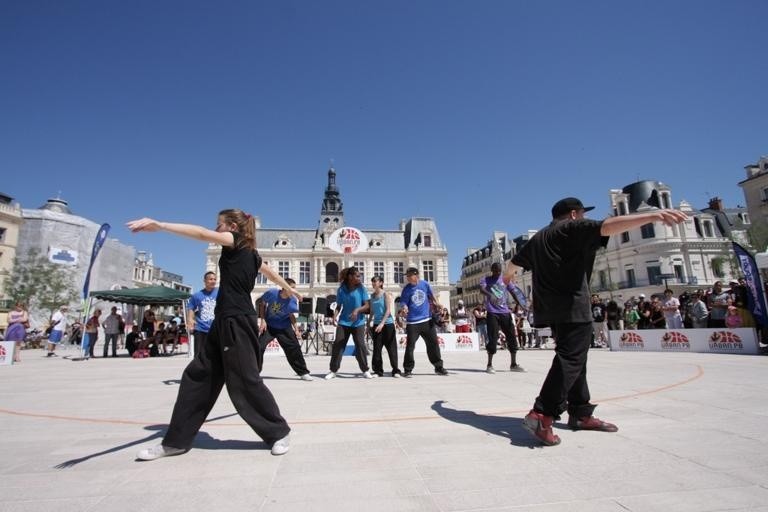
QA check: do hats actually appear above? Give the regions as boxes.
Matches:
[403,267,418,275]
[551,198,596,217]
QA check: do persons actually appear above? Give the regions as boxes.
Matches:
[501,196,689,446]
[81,299,190,361]
[187,271,220,360]
[256,278,313,381]
[368,275,402,378]
[399,267,449,376]
[433,299,506,350]
[45,305,68,356]
[588,275,759,348]
[122,207,307,462]
[308,300,410,351]
[5,302,28,363]
[508,298,558,349]
[322,266,373,378]
[478,262,529,373]
[70,319,84,346]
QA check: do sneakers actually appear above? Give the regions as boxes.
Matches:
[301,365,524,381]
[136,443,187,460]
[271,434,291,455]
[522,409,561,446]
[567,415,618,432]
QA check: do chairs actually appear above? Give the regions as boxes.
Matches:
[167,339,181,353]
[153,342,163,354]
